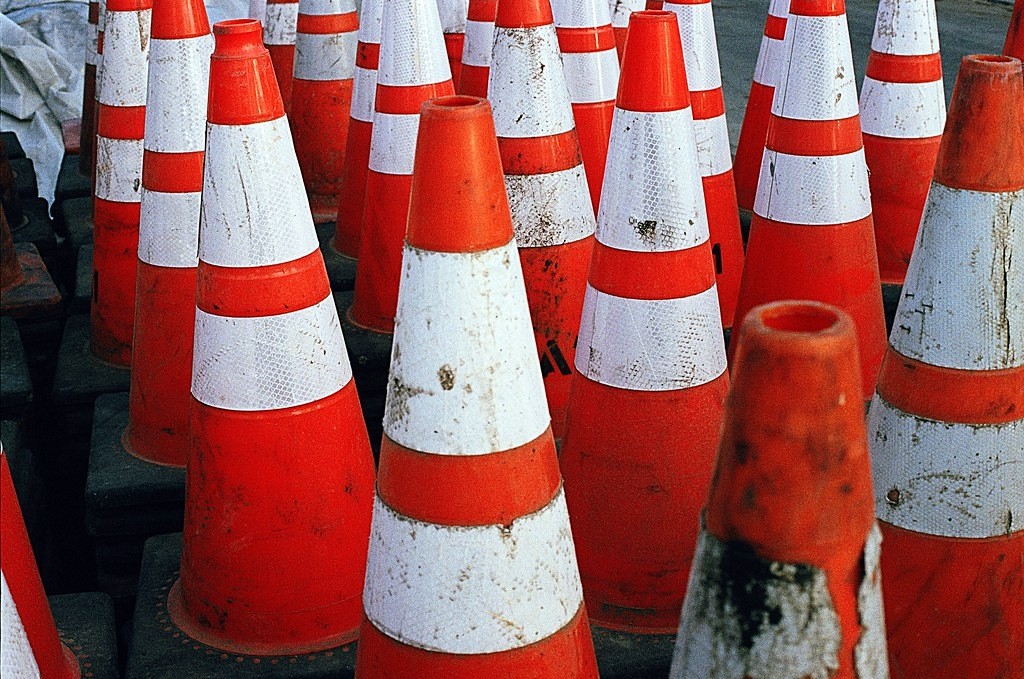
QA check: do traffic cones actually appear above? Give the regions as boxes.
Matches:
[0,440,83,679]
[555,6,735,673]
[53,2,652,509]
[320,87,604,673]
[119,16,375,678]
[987,4,1024,70]
[862,46,1022,679]
[666,291,896,679]
[858,0,951,321]
[648,1,743,339]
[723,0,894,412]
